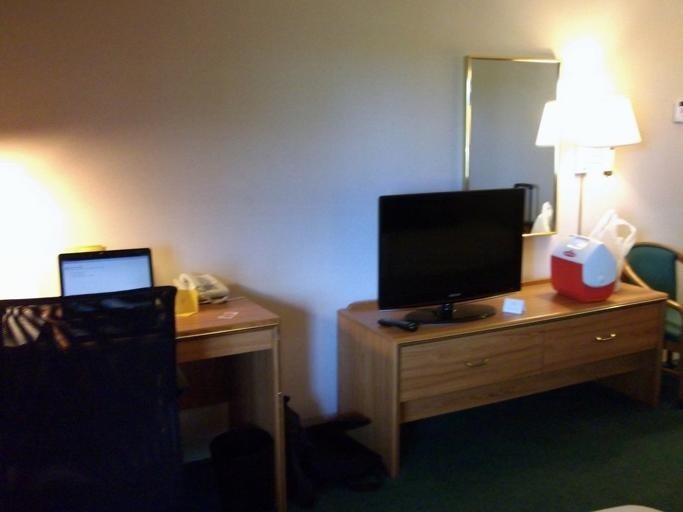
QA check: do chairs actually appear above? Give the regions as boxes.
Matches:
[622,240,683,408]
[0,285,177,512]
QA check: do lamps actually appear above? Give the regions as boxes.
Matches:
[534,95,642,236]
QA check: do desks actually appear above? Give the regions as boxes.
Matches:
[338,278,668,477]
[0,294,289,512]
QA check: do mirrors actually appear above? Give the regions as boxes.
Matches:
[464,54,561,237]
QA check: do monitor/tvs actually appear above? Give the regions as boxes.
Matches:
[379,187,526,326]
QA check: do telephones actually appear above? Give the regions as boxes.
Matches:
[173,272,230,304]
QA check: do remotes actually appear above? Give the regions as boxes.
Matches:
[377,318,417,334]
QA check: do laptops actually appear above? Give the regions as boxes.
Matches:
[61,247,155,298]
